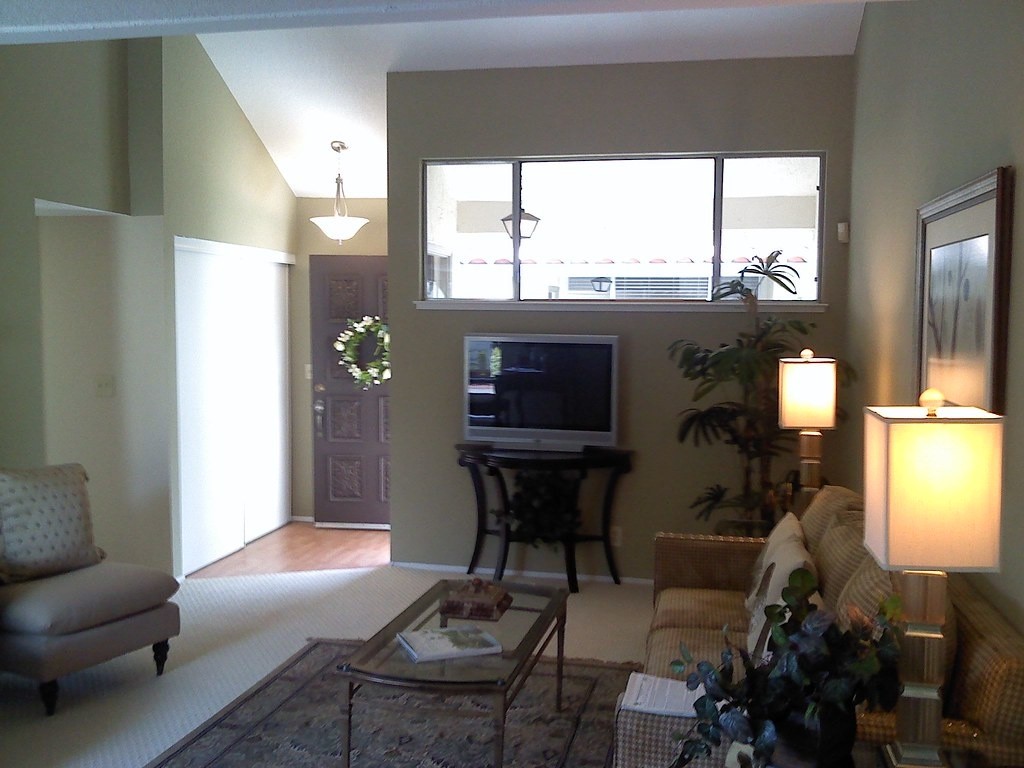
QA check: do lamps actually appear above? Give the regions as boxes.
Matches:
[308,140,370,246]
[591,277,613,292]
[860,388,1002,768]
[777,349,838,486]
[501,208,540,240]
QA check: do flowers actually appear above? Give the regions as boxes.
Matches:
[333,315,392,390]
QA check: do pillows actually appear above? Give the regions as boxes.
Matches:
[744,511,805,611]
[747,533,818,661]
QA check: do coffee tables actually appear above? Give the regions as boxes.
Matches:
[332,580,570,768]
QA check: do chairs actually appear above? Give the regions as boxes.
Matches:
[0,464,181,714]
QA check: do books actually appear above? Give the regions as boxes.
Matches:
[396,624,502,662]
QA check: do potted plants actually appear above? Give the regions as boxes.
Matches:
[664,247,854,535]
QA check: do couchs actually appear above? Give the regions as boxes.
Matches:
[615,485,1024,768]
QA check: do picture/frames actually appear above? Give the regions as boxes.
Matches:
[913,165,1013,415]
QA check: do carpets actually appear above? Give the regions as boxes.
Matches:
[142,637,642,768]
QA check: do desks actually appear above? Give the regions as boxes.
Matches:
[452,443,635,594]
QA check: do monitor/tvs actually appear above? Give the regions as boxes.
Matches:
[461,333,618,454]
